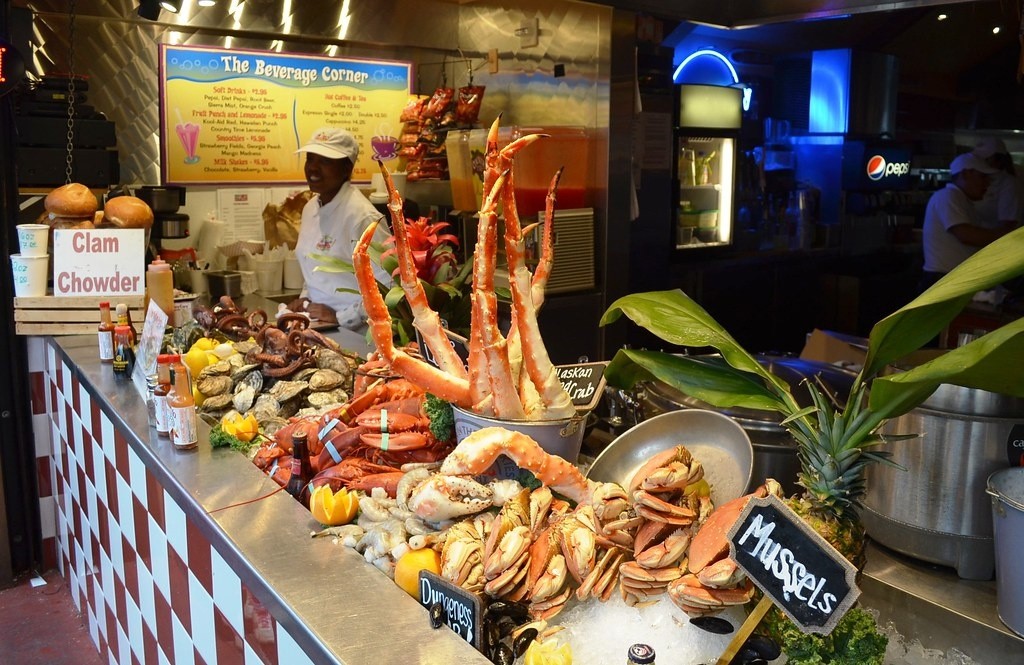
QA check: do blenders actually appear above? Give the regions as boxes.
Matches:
[444,123,595,295]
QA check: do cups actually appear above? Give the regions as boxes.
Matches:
[189,268,210,297]
[248,253,304,291]
[9,224,49,296]
[762,118,792,144]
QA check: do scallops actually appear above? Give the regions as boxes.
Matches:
[196,342,357,461]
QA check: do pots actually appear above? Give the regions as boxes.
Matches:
[618,333,1024,639]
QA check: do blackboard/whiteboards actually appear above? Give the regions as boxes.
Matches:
[733,505,850,629]
[559,364,606,406]
[419,577,476,646]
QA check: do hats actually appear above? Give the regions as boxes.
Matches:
[974,135,1007,155]
[950,153,998,176]
[295,128,360,166]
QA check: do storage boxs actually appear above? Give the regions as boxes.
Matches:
[801,328,869,372]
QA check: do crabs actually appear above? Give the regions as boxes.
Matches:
[205,342,457,515]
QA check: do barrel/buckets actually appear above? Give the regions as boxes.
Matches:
[450,401,592,492]
[376,173,407,202]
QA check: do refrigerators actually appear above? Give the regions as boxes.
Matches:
[627,81,745,319]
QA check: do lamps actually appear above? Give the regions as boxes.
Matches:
[135,0,218,21]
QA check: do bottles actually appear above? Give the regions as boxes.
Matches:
[112,303,136,382]
[98,302,114,364]
[681,148,695,186]
[146,355,198,451]
[695,152,709,185]
[286,431,312,510]
[146,255,175,326]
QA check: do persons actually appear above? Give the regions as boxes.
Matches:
[921,151,1015,289]
[966,138,1022,232]
[286,128,397,337]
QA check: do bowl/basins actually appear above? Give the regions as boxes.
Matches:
[585,408,755,506]
[369,193,387,204]
[677,208,718,245]
